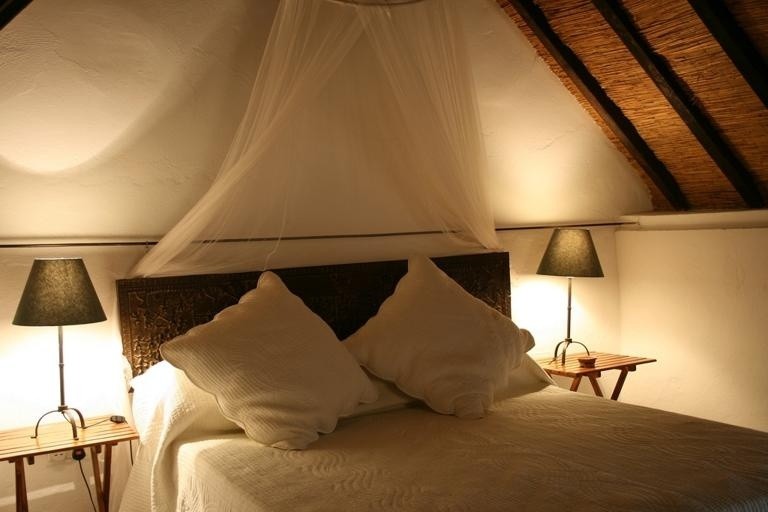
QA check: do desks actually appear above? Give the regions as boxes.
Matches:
[536,350,658,401]
[0,414,140,512]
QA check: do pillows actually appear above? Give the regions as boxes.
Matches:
[132,358,555,453]
[340,252,534,424]
[157,268,379,451]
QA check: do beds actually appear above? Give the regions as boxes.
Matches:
[115,249,768,512]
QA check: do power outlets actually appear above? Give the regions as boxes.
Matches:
[47,451,67,464]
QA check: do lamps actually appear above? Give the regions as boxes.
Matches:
[534,229,605,366]
[12,258,108,441]
